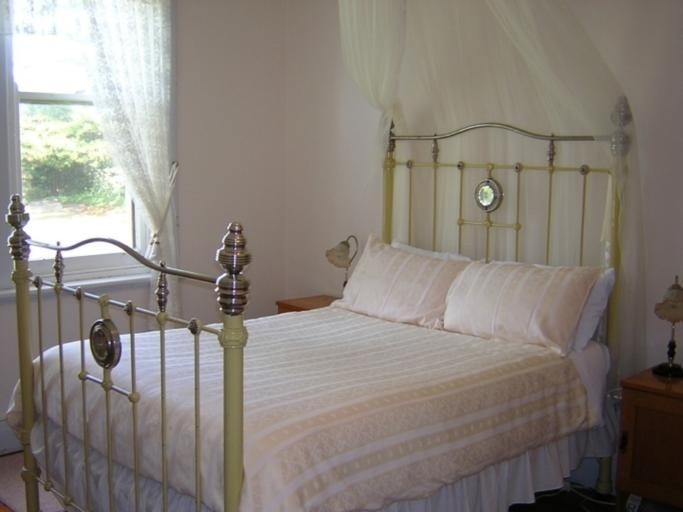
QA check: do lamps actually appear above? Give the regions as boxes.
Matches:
[650,275,683,379]
[325,235,359,298]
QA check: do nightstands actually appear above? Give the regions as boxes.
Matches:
[616,369,683,509]
[275,295,340,314]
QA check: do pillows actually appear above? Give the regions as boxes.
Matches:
[330,233,617,358]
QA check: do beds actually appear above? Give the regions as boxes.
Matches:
[5,95,632,512]
